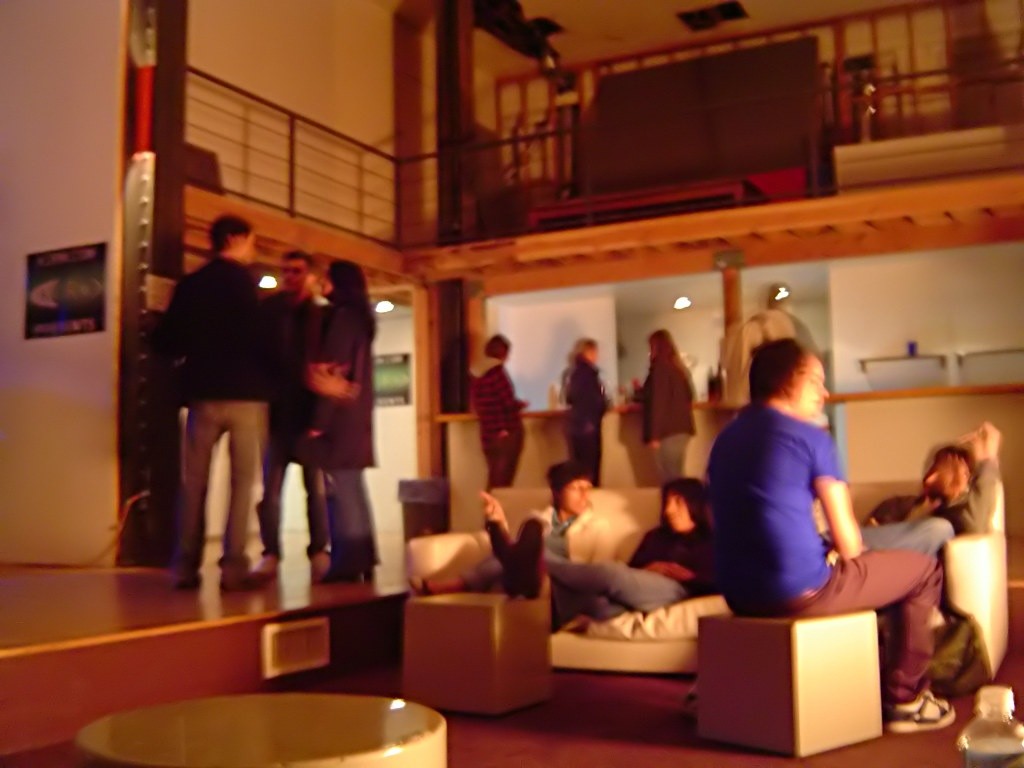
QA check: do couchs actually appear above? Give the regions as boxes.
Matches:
[408,481,1009,701]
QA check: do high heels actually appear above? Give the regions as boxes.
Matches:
[323,560,374,585]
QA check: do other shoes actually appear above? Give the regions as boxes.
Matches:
[517,517,547,599]
[310,553,331,584]
[174,575,201,590]
[489,521,517,597]
[220,572,264,593]
[252,554,279,576]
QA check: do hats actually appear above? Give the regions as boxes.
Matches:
[546,463,593,494]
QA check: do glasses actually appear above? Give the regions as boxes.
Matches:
[283,266,302,275]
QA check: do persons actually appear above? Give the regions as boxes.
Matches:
[407,421,1002,633]
[149,214,379,590]
[558,339,611,486]
[472,334,531,492]
[707,340,953,735]
[619,330,694,487]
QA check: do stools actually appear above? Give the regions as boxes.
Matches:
[74,692,448,768]
[401,590,553,716]
[699,612,883,756]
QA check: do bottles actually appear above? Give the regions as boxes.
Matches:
[955,685,1024,768]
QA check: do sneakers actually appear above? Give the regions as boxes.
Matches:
[888,689,956,733]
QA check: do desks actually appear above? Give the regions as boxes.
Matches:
[435,382,1024,585]
